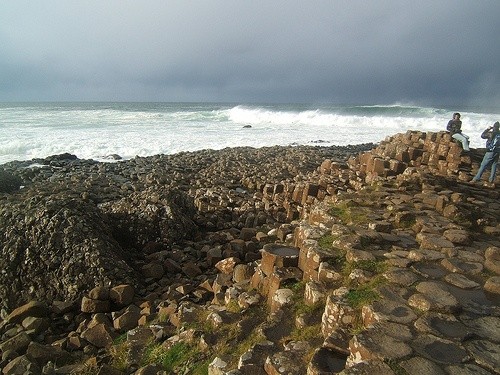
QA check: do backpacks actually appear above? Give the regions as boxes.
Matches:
[487,131,500,153]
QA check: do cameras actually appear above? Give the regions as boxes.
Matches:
[491,127,493,129]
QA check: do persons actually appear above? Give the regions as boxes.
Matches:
[470,121,500,183]
[447,112,471,153]
